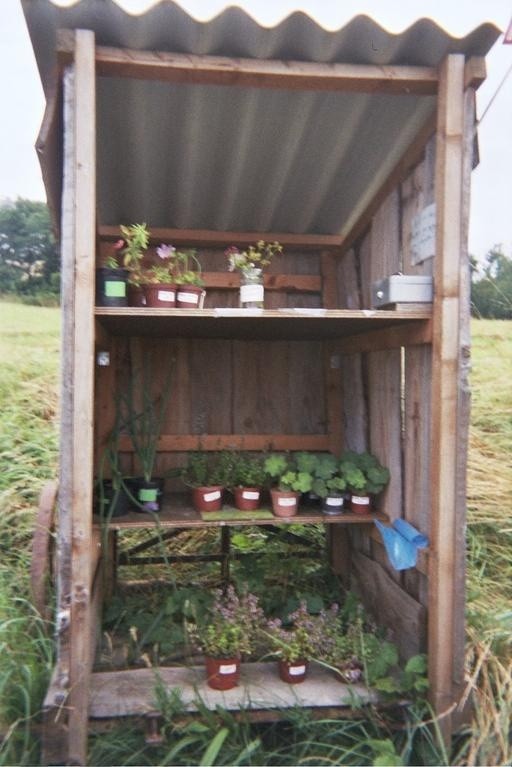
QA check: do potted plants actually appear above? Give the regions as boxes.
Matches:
[313,453,347,515]
[339,453,391,514]
[95,388,159,517]
[228,456,266,511]
[182,442,232,512]
[265,451,318,517]
[117,341,177,514]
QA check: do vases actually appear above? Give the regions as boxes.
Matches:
[127,288,148,306]
[277,658,309,684]
[144,283,178,306]
[204,655,240,690]
[240,278,264,312]
[175,286,203,307]
[335,663,365,684]
[97,268,129,307]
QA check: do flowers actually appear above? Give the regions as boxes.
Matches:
[158,244,204,284]
[103,222,149,283]
[190,582,264,658]
[266,602,312,659]
[225,240,283,278]
[312,604,395,666]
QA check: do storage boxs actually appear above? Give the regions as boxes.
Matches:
[371,274,434,309]
